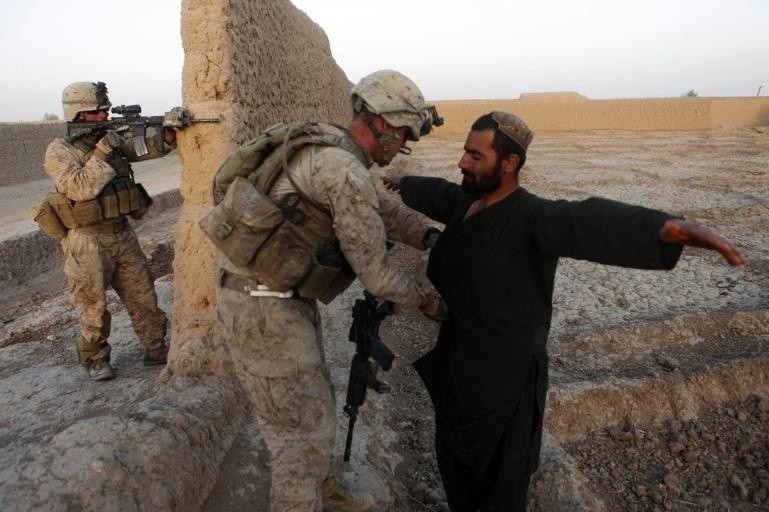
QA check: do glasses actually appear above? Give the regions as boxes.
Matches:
[84,108,111,115]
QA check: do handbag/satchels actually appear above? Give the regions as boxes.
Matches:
[198,175,285,268]
[30,197,70,244]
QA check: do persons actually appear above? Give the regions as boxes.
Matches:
[199,69,443,511]
[42,82,176,381]
[380,111,748,512]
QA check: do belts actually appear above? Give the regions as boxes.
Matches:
[218,266,274,297]
[77,217,131,235]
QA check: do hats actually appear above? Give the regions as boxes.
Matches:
[487,110,535,152]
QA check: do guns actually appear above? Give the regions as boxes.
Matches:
[343,241,397,462]
[66,105,220,157]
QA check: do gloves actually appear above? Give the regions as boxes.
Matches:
[161,107,191,132]
[424,228,444,256]
[420,288,453,326]
[94,131,125,156]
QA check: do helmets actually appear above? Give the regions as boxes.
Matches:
[61,82,112,124]
[349,69,445,143]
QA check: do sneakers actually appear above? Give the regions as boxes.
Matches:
[142,341,171,367]
[86,356,117,381]
